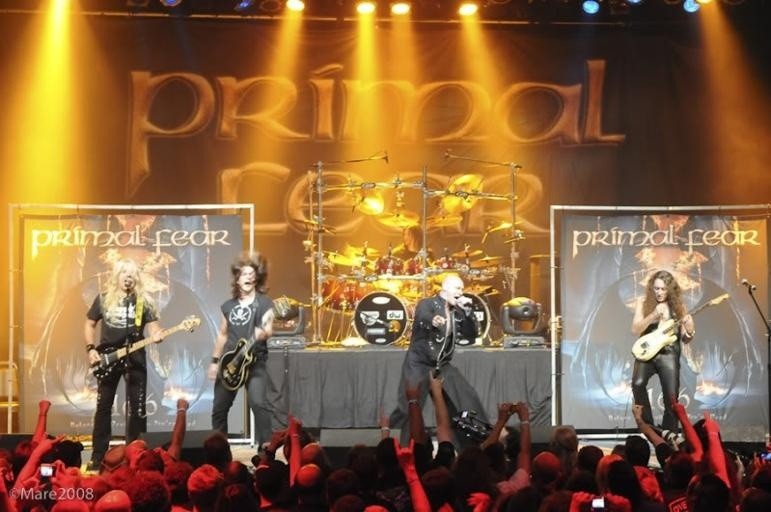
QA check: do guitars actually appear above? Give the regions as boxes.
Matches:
[91,316,201,379]
[219,298,290,391]
[631,294,729,362]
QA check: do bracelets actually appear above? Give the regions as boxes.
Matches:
[210,356,219,364]
[85,343,96,352]
[686,331,696,339]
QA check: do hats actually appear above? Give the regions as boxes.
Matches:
[58,438,84,461]
[100,444,130,471]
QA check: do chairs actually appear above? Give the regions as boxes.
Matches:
[0,360,19,432]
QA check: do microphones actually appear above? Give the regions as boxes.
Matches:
[742,279,757,291]
[126,282,131,292]
[455,297,474,309]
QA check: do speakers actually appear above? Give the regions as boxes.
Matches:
[2,435,61,469]
[319,429,410,469]
[507,427,575,461]
[137,429,221,468]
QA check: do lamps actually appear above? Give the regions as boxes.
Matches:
[498,296,546,348]
[262,295,307,350]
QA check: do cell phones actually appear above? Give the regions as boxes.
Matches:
[40,463,56,477]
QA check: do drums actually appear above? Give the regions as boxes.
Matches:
[454,292,491,345]
[323,275,357,315]
[353,290,413,347]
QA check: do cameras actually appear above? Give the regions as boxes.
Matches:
[755,448,771,464]
[510,405,520,412]
[592,495,605,512]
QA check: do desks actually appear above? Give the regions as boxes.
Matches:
[247,338,552,446]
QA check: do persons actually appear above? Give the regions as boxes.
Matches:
[632,271,696,452]
[391,225,435,268]
[206,252,275,453]
[398,275,490,450]
[85,258,164,474]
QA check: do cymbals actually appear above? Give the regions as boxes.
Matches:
[452,250,482,257]
[425,211,462,228]
[444,174,484,213]
[351,246,377,254]
[479,256,499,260]
[489,222,520,233]
[379,209,420,227]
[354,188,383,215]
[295,219,335,230]
[326,251,346,257]
